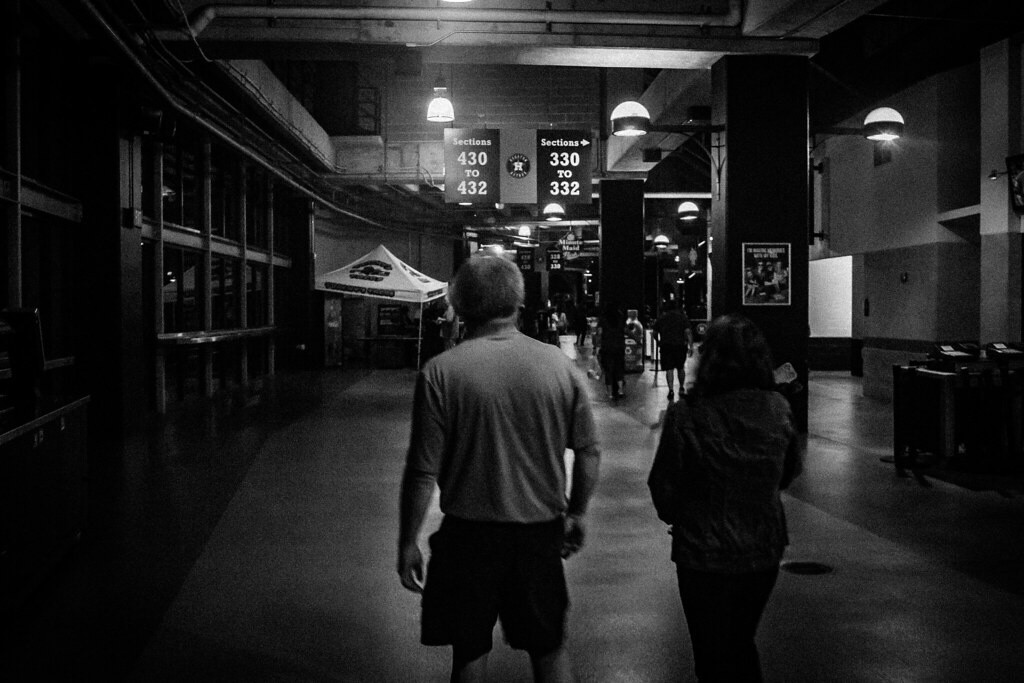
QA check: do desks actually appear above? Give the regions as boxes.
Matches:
[365,336,423,369]
[893,361,1024,490]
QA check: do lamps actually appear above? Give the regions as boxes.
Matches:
[988,168,1007,180]
[863,105,904,141]
[655,234,669,249]
[426,65,455,122]
[517,225,532,237]
[686,271,697,279]
[677,277,686,284]
[610,100,720,201]
[543,203,566,224]
[677,199,702,222]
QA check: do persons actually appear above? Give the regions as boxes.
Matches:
[745,261,789,301]
[398,255,605,683]
[418,285,697,402]
[646,314,802,683]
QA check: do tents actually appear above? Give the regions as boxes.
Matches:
[314,244,450,371]
